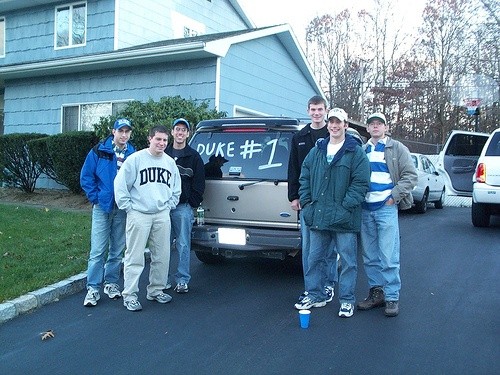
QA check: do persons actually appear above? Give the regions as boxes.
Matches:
[162,118,204,295]
[294,107,370,317]
[287,96,338,304]
[80,118,137,307]
[358,112,418,316]
[114,126,183,311]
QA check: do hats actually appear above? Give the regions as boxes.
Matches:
[328,108,347,122]
[367,112,386,125]
[114,118,133,131]
[172,117,190,131]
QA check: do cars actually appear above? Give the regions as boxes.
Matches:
[407,153,445,212]
[438,128,500,230]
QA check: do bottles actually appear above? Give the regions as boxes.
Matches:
[196,207,205,225]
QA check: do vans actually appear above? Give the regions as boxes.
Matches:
[186,117,368,272]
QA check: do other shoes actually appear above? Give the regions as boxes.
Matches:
[173,283,189,292]
[294,296,327,309]
[339,302,355,317]
[384,300,399,316]
[104,282,122,298]
[83,286,100,306]
[123,299,143,311]
[357,287,385,311]
[299,286,335,302]
[146,292,173,303]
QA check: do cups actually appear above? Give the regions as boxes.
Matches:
[299,309,311,329]
